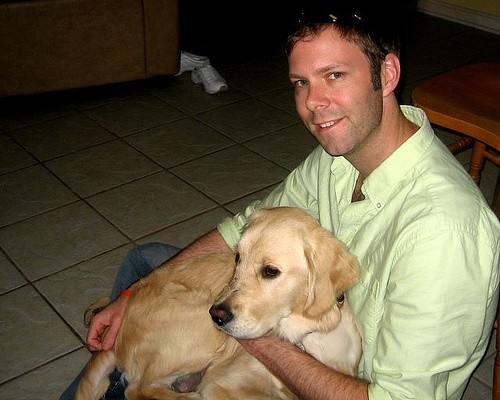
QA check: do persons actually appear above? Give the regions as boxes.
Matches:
[55,1,500,400]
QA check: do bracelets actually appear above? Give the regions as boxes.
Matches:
[118,289,133,298]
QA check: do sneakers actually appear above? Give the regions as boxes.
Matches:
[175,51,210,77]
[191,64,228,95]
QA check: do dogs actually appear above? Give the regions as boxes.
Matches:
[73,206,363,400]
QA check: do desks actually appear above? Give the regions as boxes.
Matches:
[413,59,499,195]
